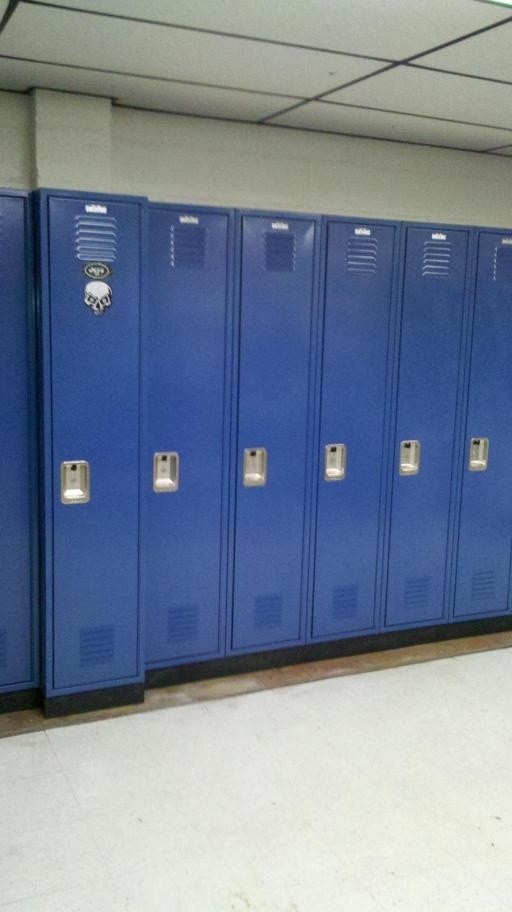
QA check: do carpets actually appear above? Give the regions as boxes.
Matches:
[1,644,507,912]
